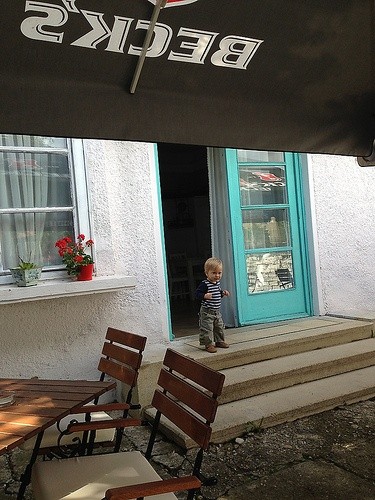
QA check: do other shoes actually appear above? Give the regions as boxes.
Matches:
[205,346,217,353]
[215,342,229,348]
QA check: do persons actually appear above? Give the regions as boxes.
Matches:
[197,258,230,353]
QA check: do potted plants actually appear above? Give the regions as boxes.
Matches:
[8,252,45,288]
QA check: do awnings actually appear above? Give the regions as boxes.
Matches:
[0,0,375,167]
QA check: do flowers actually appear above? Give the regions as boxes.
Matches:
[54,234,95,276]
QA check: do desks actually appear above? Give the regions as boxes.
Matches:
[0,378,117,500]
[170,257,202,301]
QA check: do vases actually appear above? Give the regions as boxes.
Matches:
[75,264,93,281]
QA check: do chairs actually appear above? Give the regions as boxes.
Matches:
[167,250,196,300]
[17,326,226,500]
